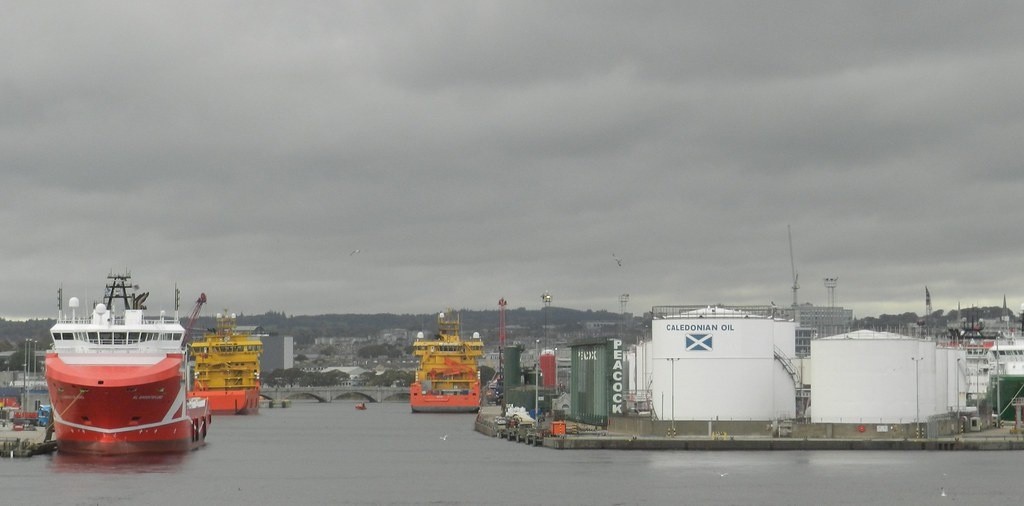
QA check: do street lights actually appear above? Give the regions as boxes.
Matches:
[912,357,924,439]
[667,358,680,437]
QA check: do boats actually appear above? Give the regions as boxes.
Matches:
[43,269,212,455]
[411,309,484,414]
[189,311,264,415]
[355,403,366,410]
[269,399,291,409]
